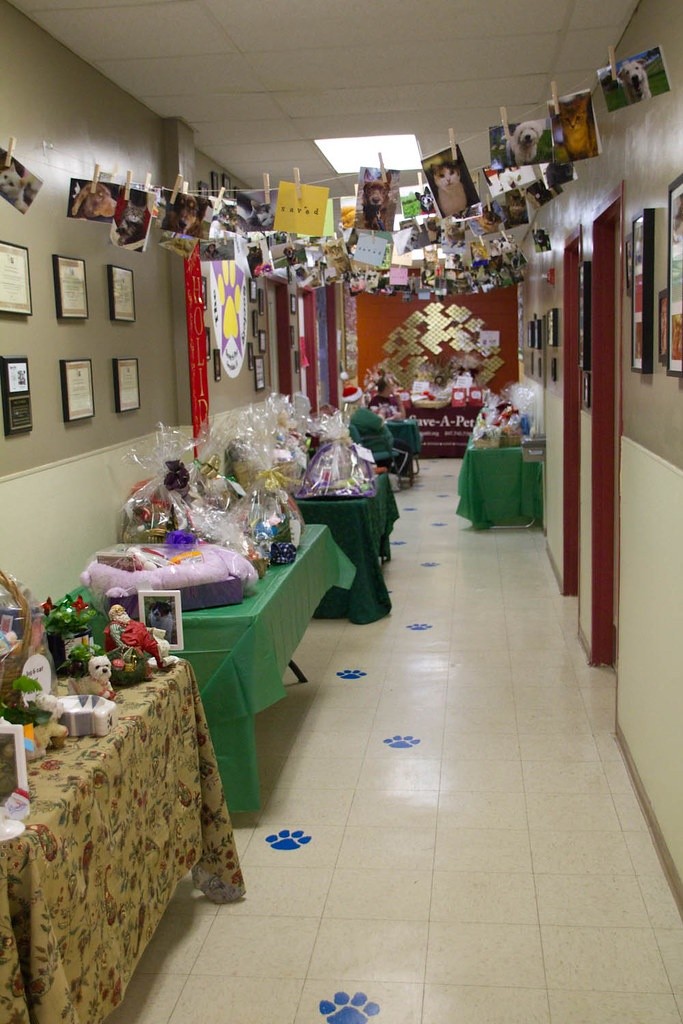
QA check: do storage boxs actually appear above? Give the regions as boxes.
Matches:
[105,574,243,619]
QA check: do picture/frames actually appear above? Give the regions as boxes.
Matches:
[139,591,184,650]
[247,276,266,391]
[0,241,144,436]
[527,174,683,408]
[205,326,211,356]
[289,293,300,373]
[213,349,221,381]
[200,276,207,309]
[0,725,27,797]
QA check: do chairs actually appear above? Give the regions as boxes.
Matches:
[350,425,408,490]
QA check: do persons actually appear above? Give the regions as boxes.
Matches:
[104,605,165,671]
[368,379,406,426]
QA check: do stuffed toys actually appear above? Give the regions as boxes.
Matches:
[68,653,116,700]
[127,546,167,570]
[2,692,69,761]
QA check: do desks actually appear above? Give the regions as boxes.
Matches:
[0,403,543,1015]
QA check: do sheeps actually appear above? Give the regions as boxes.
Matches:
[67,654,114,701]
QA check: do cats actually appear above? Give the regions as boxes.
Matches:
[507,194,526,215]
[430,160,467,215]
[617,60,656,105]
[558,95,593,160]
[0,162,28,210]
[246,200,274,226]
[512,121,543,163]
